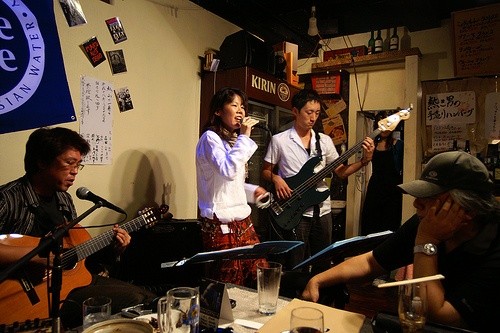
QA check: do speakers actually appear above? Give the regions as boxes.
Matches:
[118,218,204,294]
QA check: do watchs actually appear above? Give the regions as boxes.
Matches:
[413,243,437,255]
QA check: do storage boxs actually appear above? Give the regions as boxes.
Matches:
[273,42,298,72]
[323,45,368,61]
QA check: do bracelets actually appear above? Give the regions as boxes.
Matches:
[360,158,369,166]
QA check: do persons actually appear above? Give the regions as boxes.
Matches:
[0,127,131,267]
[196,86,269,286]
[358,111,404,257]
[261,88,375,302]
[300,151,500,333]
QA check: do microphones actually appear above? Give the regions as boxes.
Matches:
[241,117,269,131]
[76,186,127,215]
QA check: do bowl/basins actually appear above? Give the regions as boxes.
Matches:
[81,318,154,333]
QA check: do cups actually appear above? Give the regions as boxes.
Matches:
[158,286,199,333]
[256,261,282,317]
[398,283,427,333]
[82,295,112,331]
[290,307,324,333]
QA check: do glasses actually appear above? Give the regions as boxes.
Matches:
[52,155,86,172]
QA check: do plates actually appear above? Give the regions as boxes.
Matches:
[132,312,158,333]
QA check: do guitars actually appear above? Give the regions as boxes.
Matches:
[0,204,169,325]
[265,107,410,231]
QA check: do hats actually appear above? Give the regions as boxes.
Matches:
[395,149,493,198]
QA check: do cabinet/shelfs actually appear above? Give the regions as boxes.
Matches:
[200,66,304,239]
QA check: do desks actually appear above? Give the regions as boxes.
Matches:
[63,282,375,333]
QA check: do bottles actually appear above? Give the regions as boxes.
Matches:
[368,28,375,55]
[374,27,383,53]
[465,139,471,154]
[453,138,457,151]
[389,27,399,51]
[476,153,500,204]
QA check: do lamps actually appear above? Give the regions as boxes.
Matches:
[307,6,318,37]
